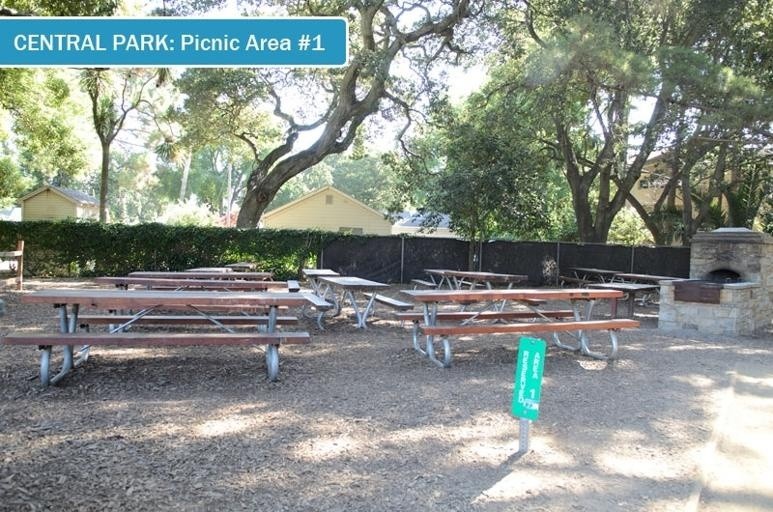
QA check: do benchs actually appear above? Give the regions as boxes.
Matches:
[2,262,675,392]
